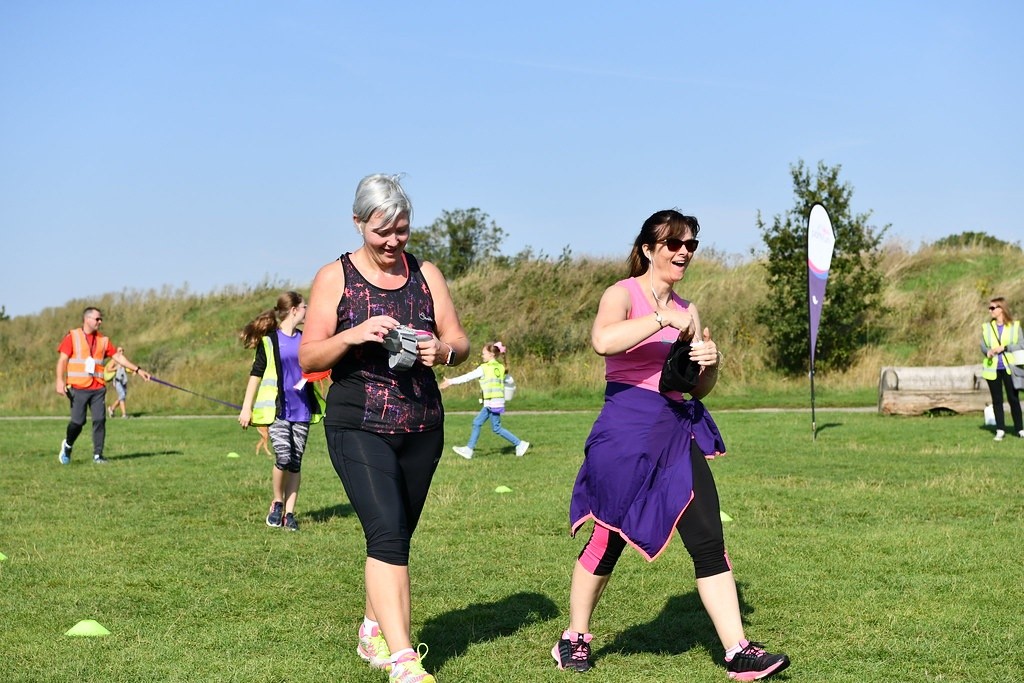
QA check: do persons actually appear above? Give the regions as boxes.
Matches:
[108,346,130,418]
[238,291,326,530]
[550,209,791,683]
[440,342,530,459]
[56,308,151,465]
[297,173,470,683]
[980,297,1024,441]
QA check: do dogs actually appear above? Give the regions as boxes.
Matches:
[255,425,273,456]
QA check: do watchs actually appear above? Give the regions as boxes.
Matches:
[443,343,456,366]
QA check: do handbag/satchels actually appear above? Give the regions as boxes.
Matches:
[1009,365,1024,389]
[504,386,514,401]
[104,362,116,381]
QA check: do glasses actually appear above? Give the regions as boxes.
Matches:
[659,238,699,252]
[91,317,104,322]
[989,306,1000,311]
[297,305,308,310]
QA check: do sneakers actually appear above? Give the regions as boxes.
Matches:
[452,446,473,459]
[266,501,285,527]
[122,416,129,419]
[93,456,108,463]
[283,513,297,531]
[59,439,72,464]
[357,623,392,672]
[389,643,436,683]
[993,430,1005,440]
[551,630,594,673]
[107,407,114,417]
[1017,430,1024,438]
[516,440,530,457]
[724,640,791,681]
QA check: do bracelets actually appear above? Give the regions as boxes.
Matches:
[654,311,664,329]
[136,366,141,373]
